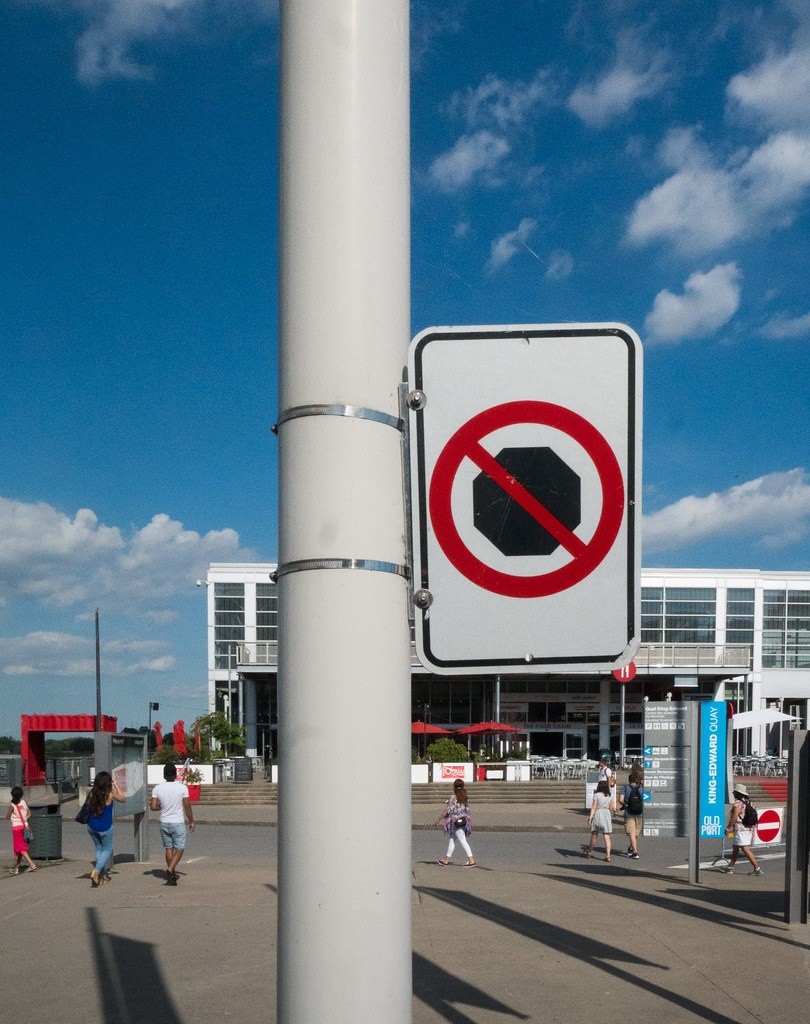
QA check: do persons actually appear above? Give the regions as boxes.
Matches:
[719,783,763,876]
[436,779,476,868]
[598,759,611,785]
[150,763,195,884]
[619,774,644,859]
[7,787,38,875]
[87,771,125,886]
[584,781,614,861]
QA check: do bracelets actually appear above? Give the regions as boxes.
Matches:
[726,828,729,831]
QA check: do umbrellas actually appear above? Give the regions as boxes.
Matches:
[454,721,521,735]
[411,720,452,734]
[153,720,201,753]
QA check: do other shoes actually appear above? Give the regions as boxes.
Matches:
[748,867,763,876]
[632,853,640,859]
[163,869,177,886]
[627,847,633,857]
[9,868,19,874]
[602,856,610,862]
[720,866,734,875]
[90,868,111,887]
[27,865,37,872]
[463,860,476,868]
[583,850,592,858]
[438,859,448,867]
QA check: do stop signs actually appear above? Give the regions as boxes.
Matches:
[752,805,786,847]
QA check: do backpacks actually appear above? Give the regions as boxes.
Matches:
[624,785,643,815]
[738,798,757,828]
[600,767,615,788]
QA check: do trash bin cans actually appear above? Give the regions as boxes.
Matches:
[26,804,63,859]
[586,771,617,810]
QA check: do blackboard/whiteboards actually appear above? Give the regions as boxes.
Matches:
[235,759,253,781]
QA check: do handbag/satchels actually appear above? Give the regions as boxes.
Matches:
[454,817,467,827]
[75,789,93,824]
[24,825,34,844]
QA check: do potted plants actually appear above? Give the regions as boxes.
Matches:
[475,755,486,781]
[182,766,203,803]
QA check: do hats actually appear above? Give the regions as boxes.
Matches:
[732,784,749,796]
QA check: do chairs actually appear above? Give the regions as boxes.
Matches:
[734,755,788,777]
[528,756,595,780]
